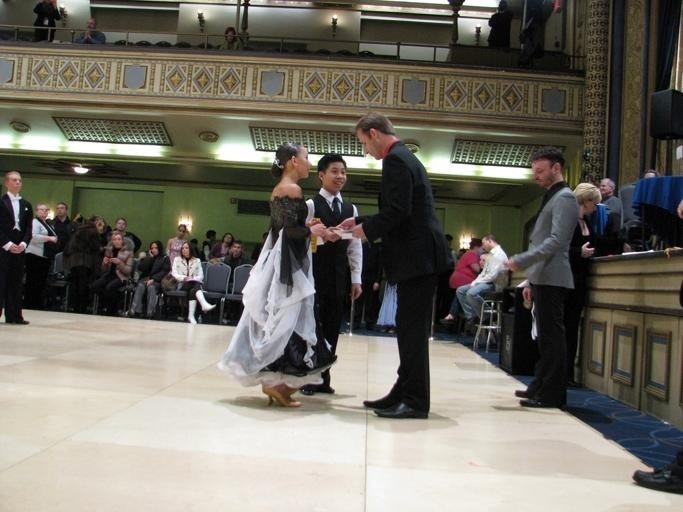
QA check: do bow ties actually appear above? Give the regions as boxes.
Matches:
[11,195,22,201]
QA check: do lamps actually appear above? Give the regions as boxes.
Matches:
[476,24,481,46]
[330,15,338,37]
[198,11,206,32]
[59,6,67,28]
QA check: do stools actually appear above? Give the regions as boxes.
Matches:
[474,294,501,353]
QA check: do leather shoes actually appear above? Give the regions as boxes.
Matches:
[301,385,313,395]
[514,389,530,398]
[314,383,335,393]
[363,394,395,409]
[520,398,561,408]
[374,401,429,420]
[5,318,30,325]
[633,463,672,482]
[638,470,683,495]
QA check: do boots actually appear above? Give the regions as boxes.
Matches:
[187,300,197,324]
[196,290,216,312]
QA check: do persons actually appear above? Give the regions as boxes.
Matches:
[565,182,602,388]
[76,19,106,45]
[351,237,383,331]
[251,232,269,260]
[172,241,216,324]
[457,233,509,326]
[355,113,448,421]
[33,0,61,42]
[645,169,658,178]
[487,1,511,69]
[128,241,171,320]
[440,252,489,324]
[0,171,33,324]
[444,233,457,271]
[217,142,338,407]
[449,237,481,293]
[112,217,142,253]
[165,224,188,266]
[632,200,683,494]
[210,240,251,293]
[23,204,58,309]
[208,233,234,260]
[64,221,100,315]
[92,232,133,316]
[503,147,580,407]
[300,154,362,395]
[48,201,78,280]
[599,178,623,232]
[218,26,243,51]
[93,217,113,246]
[203,230,215,263]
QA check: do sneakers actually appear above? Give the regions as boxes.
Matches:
[379,326,388,333]
[439,318,457,324]
[388,328,397,334]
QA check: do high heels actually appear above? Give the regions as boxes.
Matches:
[261,384,303,407]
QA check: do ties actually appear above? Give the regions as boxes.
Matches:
[333,199,340,221]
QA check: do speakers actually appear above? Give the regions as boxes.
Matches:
[497,287,534,375]
[648,89,683,139]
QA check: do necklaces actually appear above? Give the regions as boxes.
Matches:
[577,217,588,231]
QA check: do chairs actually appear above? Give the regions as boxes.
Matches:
[164,290,187,298]
[50,251,69,310]
[107,238,134,251]
[227,264,254,301]
[203,262,231,324]
